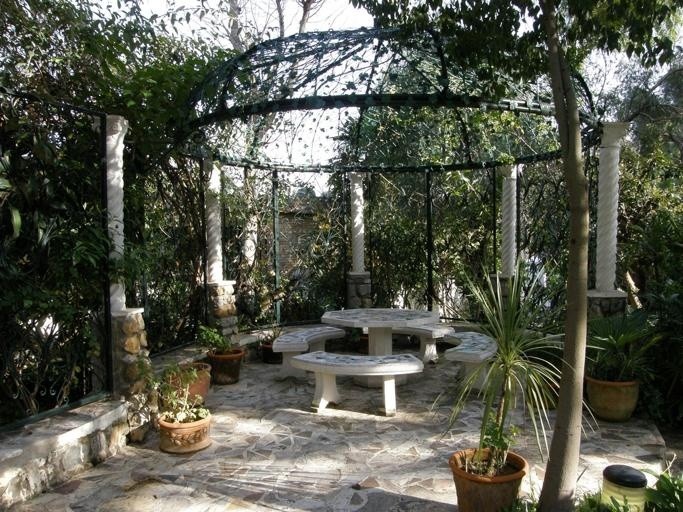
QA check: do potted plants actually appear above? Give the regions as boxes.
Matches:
[432,254,610,511]
[136,311,291,455]
[582,305,666,418]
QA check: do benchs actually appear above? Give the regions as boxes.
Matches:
[271,323,498,417]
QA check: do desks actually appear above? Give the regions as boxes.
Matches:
[320,307,439,387]
